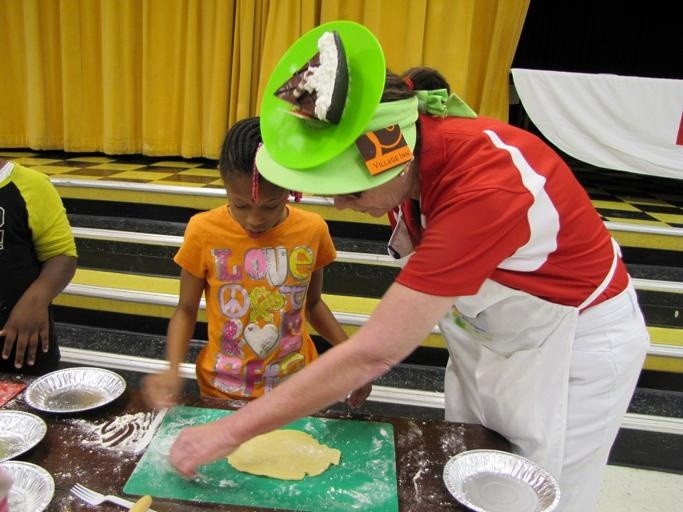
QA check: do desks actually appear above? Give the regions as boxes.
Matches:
[1,373,524,511]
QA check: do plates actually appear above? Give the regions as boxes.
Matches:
[0,410,46,462]
[0,459,55,511]
[443,447,561,511]
[24,365,128,414]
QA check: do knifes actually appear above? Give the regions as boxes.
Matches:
[132,405,169,455]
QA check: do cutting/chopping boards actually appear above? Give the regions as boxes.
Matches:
[124,403,399,511]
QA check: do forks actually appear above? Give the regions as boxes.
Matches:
[70,483,155,512]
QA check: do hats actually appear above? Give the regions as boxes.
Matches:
[254,88,478,195]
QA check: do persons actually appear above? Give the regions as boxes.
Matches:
[137,115,372,414]
[164,19,648,512]
[0,157,80,376]
